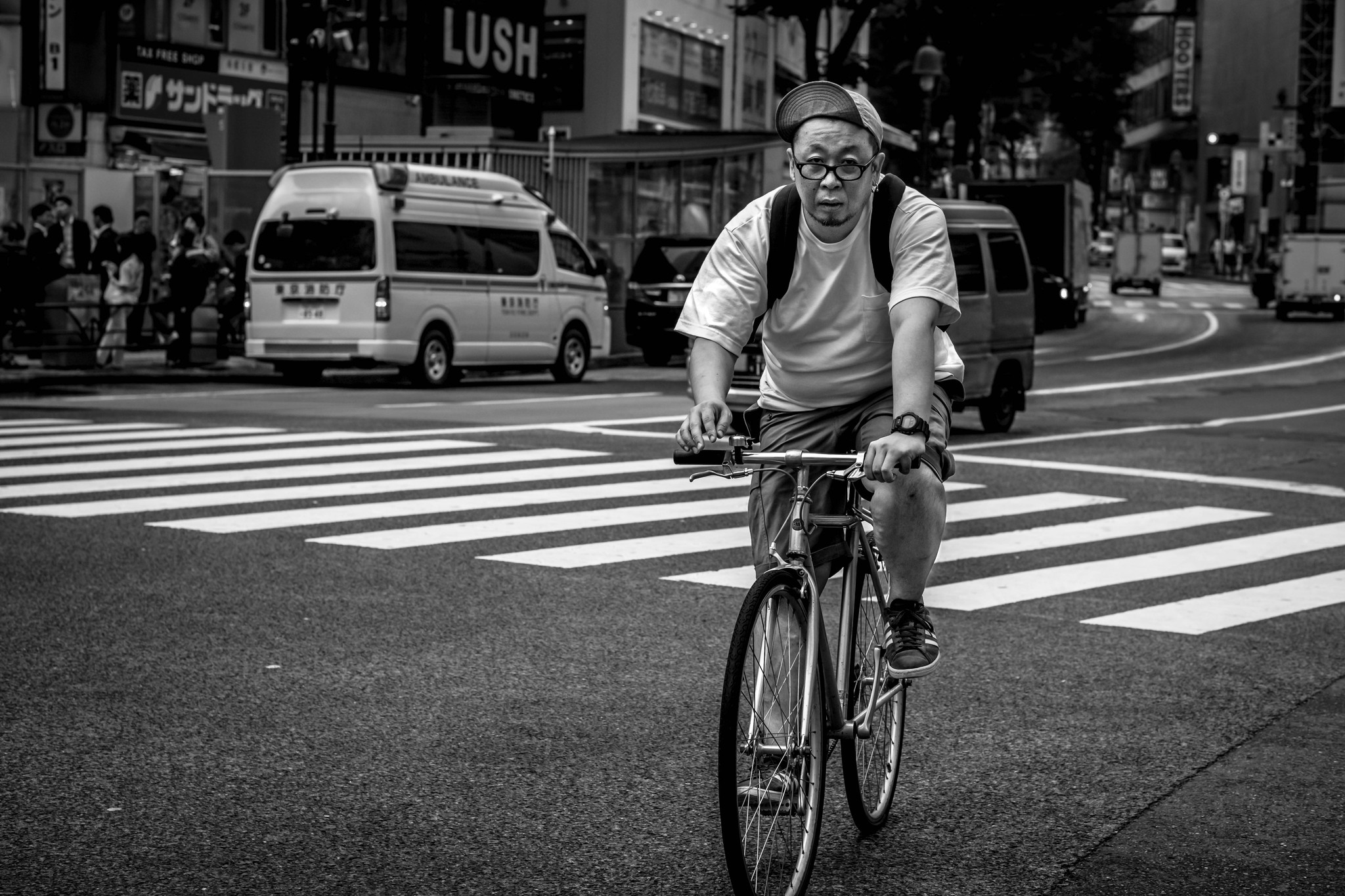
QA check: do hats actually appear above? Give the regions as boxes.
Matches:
[775,79,883,149]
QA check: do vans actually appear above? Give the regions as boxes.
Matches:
[685,198,1037,437]
[240,160,613,385]
[624,234,718,365]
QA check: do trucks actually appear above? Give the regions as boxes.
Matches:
[1111,231,1163,297]
[966,177,1095,332]
[1276,232,1345,322]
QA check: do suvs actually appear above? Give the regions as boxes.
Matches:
[1161,233,1188,274]
[1086,231,1114,266]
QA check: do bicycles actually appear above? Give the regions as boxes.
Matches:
[672,449,915,895]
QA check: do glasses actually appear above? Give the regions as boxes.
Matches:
[790,143,877,182]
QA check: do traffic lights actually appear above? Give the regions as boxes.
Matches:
[1205,132,1239,147]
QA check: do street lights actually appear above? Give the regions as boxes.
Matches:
[911,44,944,198]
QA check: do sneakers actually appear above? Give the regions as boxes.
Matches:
[737,755,810,808]
[882,598,940,680]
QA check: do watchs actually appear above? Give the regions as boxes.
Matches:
[891,412,930,442]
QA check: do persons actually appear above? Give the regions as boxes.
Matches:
[674,80,965,805]
[1210,227,1272,308]
[0,195,249,370]
